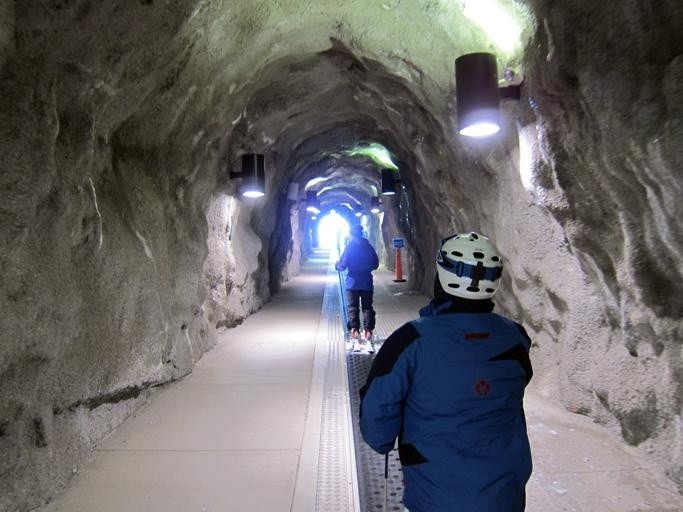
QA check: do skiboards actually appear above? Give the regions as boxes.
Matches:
[353,329,374,353]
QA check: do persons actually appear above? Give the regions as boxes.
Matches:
[334,223,378,340]
[356,229,533,511]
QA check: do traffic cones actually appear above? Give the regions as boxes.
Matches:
[393,248,406,282]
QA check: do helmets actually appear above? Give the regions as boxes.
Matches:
[437,232,503,299]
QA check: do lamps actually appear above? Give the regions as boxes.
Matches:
[370,169,400,214]
[453,53,521,138]
[229,154,265,197]
[300,190,317,211]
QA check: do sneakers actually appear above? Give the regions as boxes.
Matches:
[353,328,359,338]
[365,329,372,339]
